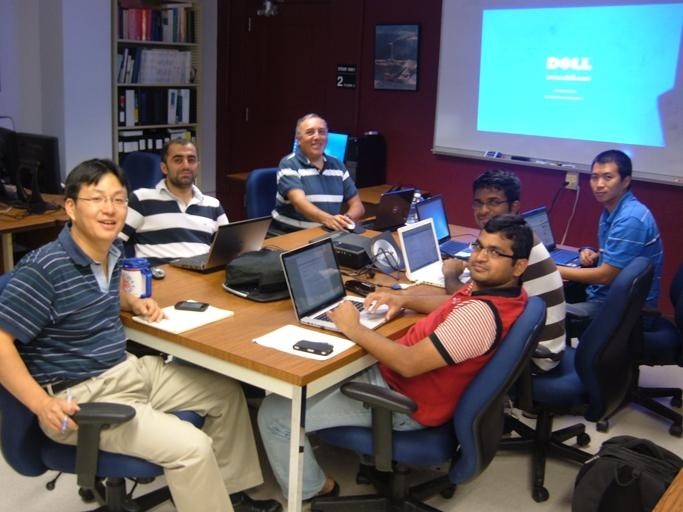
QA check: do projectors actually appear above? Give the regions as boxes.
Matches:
[310,230,373,268]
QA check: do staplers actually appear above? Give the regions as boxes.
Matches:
[344,280,376,298]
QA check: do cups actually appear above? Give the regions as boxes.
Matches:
[121,257,152,300]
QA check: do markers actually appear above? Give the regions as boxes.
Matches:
[511,156,532,161]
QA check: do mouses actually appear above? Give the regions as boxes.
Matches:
[345,219,356,230]
[152,267,166,279]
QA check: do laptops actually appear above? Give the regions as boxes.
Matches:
[414,194,473,258]
[358,188,414,234]
[520,206,584,267]
[397,216,471,288]
[168,215,271,273]
[279,237,404,332]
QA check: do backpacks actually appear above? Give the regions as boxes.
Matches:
[571,435,683,511]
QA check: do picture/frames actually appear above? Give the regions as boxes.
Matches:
[371,22,421,94]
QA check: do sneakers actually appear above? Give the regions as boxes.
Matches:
[302,477,340,499]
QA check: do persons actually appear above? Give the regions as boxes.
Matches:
[523,147,668,419]
[265,111,366,238]
[252,211,532,507]
[439,167,568,378]
[116,139,233,357]
[0,158,281,512]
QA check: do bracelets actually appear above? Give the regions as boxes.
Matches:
[343,212,353,221]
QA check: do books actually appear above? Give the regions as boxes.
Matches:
[116,3,198,85]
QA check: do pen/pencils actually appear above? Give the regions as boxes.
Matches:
[61,395,72,435]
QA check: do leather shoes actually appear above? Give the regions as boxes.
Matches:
[231,493,282,512]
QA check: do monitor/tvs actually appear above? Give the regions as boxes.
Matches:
[0,126,64,212]
[292,131,349,168]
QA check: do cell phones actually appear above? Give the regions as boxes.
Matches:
[293,339,334,357]
[175,300,209,312]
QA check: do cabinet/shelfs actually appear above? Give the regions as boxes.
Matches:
[60,1,204,185]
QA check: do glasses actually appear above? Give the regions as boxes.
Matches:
[472,198,509,209]
[73,194,129,208]
[468,240,518,260]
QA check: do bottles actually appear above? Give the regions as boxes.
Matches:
[404,192,425,226]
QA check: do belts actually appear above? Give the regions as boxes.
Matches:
[42,377,98,395]
